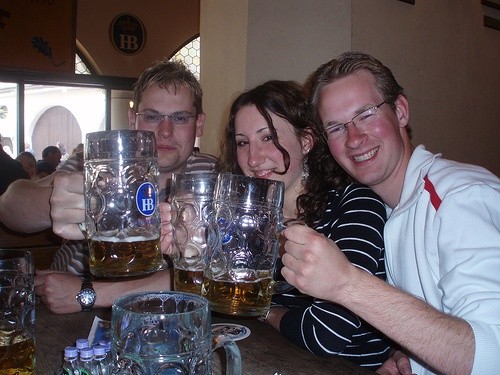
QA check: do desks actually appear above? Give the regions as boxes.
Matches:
[34,302,378,375]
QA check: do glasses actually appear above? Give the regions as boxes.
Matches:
[321,102,387,144]
[134,111,198,125]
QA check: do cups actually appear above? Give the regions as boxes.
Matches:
[112,289,252,375]
[163,156,227,300]
[0,247,38,375]
[199,170,309,316]
[82,130,163,279]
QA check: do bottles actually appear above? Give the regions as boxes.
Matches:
[63,337,115,375]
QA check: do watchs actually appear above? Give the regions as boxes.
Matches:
[75,278,96,312]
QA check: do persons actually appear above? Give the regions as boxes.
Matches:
[0,55,221,314]
[161,79,392,369]
[0,133,61,270]
[282,52,500,375]
